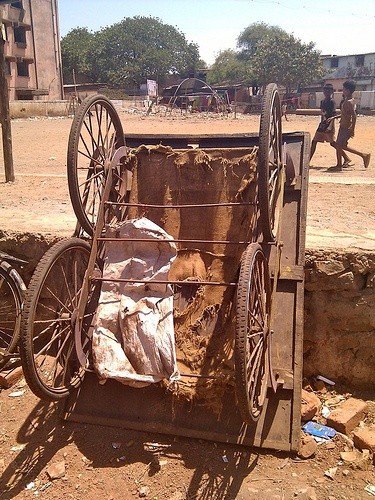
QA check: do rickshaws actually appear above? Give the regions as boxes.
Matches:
[17,81,313,453]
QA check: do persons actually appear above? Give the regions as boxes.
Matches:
[326,79,371,171]
[280,102,288,121]
[311,84,355,168]
